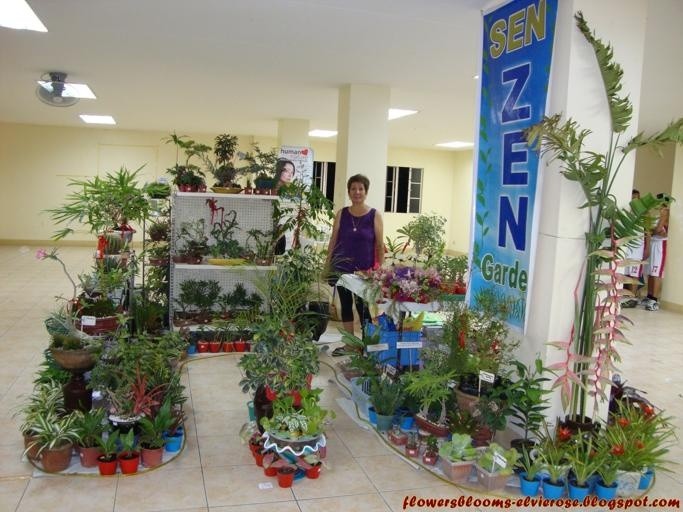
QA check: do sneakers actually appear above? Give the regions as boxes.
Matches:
[645,300,659,311]
[638,297,649,306]
[621,300,638,308]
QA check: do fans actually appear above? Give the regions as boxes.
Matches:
[36,72,80,107]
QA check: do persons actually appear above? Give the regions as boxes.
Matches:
[644,193,669,311]
[621,189,650,309]
[321,174,383,356]
[274,156,295,195]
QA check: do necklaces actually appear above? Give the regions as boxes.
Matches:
[349,207,367,232]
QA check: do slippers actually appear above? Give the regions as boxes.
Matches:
[331,347,350,357]
